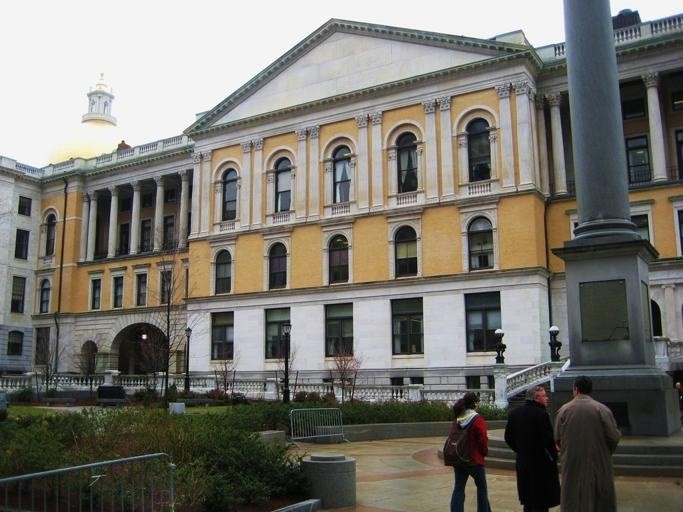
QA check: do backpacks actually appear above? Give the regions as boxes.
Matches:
[443,415,479,466]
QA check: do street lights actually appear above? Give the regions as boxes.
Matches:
[547,324,561,363]
[181,326,192,393]
[279,320,292,404]
[493,327,505,365]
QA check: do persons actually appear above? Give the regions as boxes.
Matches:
[504,386,559,512]
[553,375,620,512]
[443,392,492,511]
[675,382,683,410]
